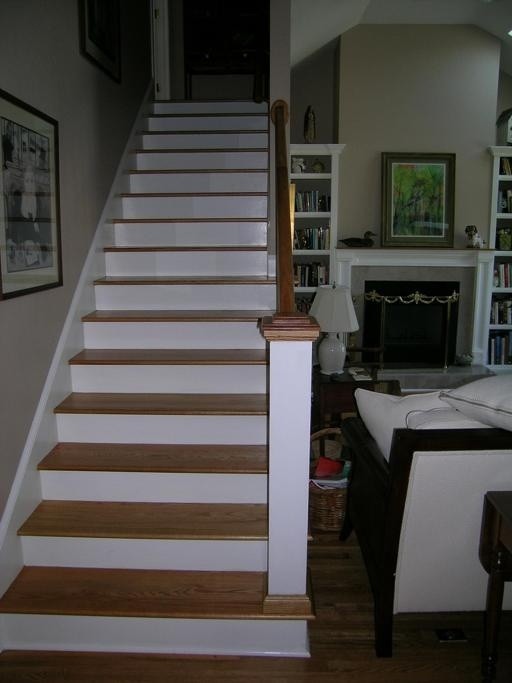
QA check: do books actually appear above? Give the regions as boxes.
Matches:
[489,156,512,366]
[310,440,350,490]
[292,190,332,287]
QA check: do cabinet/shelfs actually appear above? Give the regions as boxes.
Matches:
[291,144,346,312]
[486,145,512,374]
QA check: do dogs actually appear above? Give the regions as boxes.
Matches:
[465,225,486,249]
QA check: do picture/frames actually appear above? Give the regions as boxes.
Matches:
[77,0,122,85]
[0,88,63,302]
[380,151,456,248]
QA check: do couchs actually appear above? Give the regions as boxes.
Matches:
[339,417,512,659]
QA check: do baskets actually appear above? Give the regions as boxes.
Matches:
[310,428,353,534]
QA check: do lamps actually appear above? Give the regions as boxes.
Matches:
[308,281,360,376]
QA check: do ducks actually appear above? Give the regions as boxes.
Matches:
[338,231,377,247]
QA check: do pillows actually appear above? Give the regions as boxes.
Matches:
[439,374,512,431]
[352,387,455,463]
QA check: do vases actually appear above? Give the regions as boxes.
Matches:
[303,105,316,144]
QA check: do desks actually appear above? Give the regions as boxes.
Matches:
[312,364,375,456]
[478,491,511,683]
